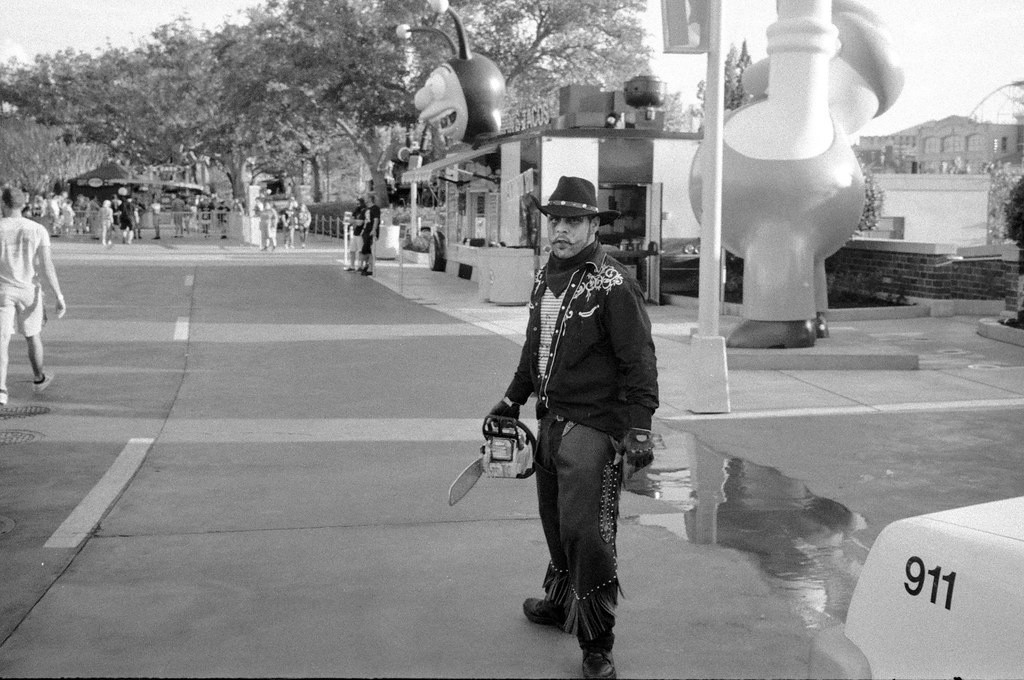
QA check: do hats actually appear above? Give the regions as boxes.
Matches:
[530,176,621,226]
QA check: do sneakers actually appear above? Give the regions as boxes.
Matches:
[33,370,55,394]
[582,649,616,680]
[523,598,573,634]
[0,388,8,405]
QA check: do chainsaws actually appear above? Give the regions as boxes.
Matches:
[448,414,537,506]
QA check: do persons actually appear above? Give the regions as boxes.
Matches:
[1,191,64,404]
[487,176,660,678]
[339,195,381,275]
[7,189,311,254]
